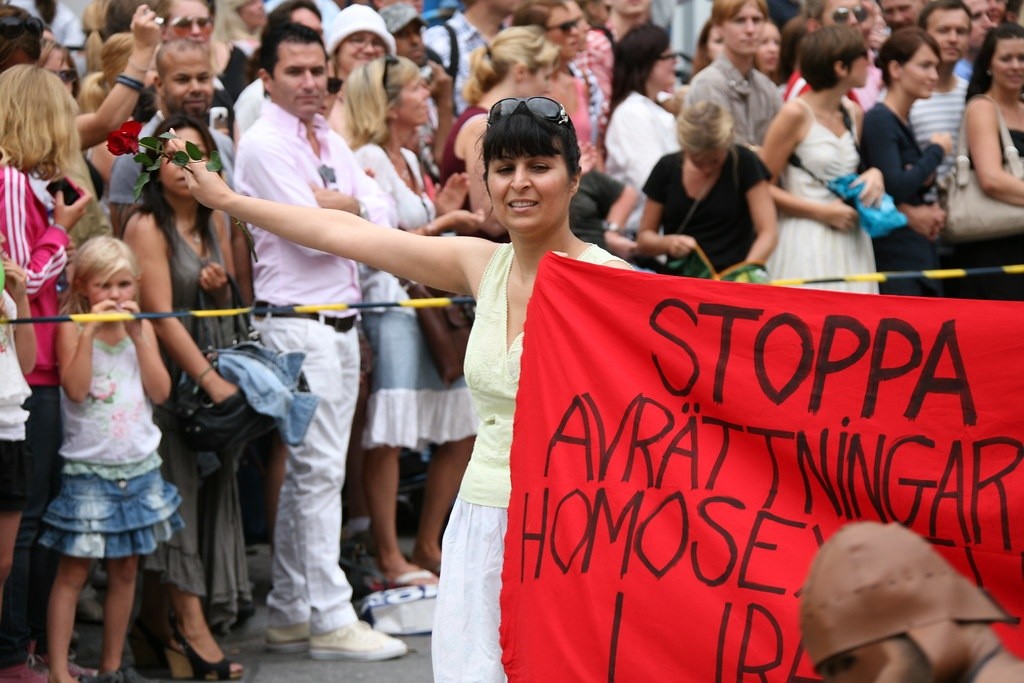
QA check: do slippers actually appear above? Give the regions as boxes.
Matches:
[376,564,438,589]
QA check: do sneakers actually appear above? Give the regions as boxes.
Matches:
[308,619,408,663]
[0,650,100,683]
[266,619,374,654]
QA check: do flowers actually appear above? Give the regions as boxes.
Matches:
[107,120,258,263]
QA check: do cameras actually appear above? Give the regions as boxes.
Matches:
[154,17,165,27]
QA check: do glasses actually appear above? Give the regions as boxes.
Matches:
[821,5,870,24]
[0,15,44,39]
[349,33,386,50]
[543,18,579,32]
[161,16,215,37]
[46,69,77,84]
[381,52,397,91]
[485,96,576,132]
[656,51,677,61]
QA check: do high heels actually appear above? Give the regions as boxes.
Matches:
[162,616,246,682]
[127,616,168,669]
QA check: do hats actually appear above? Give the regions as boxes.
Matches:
[377,2,429,35]
[323,3,398,61]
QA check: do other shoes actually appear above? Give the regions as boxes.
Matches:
[79,564,108,625]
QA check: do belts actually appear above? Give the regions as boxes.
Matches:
[252,298,359,330]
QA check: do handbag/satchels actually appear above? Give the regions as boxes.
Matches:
[403,279,479,387]
[175,269,287,448]
[694,244,773,284]
[945,93,1024,244]
[826,173,907,238]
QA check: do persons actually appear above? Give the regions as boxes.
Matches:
[186,96,633,683]
[798,522,1024,683]
[439,0,1024,301]
[0,0,475,683]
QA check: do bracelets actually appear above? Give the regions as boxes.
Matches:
[601,222,623,232]
[53,223,66,233]
[128,56,149,73]
[116,76,145,93]
[193,365,214,393]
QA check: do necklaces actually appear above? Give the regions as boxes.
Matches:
[193,235,200,243]
[386,147,411,180]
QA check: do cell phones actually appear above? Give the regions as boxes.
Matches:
[46,176,84,207]
